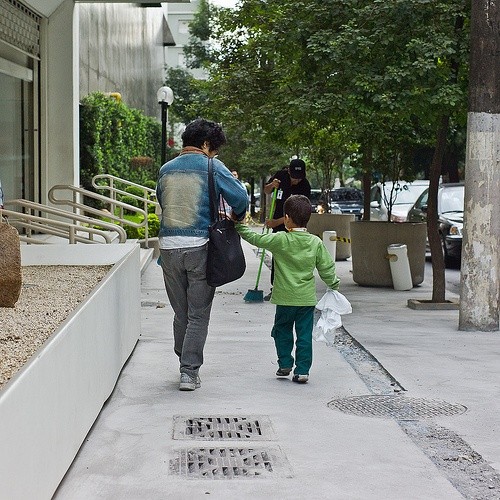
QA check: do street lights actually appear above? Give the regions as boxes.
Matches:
[157,86,175,166]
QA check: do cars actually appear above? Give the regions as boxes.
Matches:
[406,181,465,270]
[360,178,430,222]
[308,187,364,220]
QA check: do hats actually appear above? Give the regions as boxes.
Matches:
[289,159,306,179]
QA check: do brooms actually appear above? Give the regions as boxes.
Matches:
[242,187,277,302]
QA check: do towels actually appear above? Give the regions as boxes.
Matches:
[311,287,353,347]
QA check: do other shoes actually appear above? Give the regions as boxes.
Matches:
[263,288,272,301]
[276,367,292,376]
[179,372,202,390]
[292,375,308,383]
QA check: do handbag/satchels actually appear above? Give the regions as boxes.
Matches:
[205,219,246,288]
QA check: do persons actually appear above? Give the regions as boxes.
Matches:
[235,195,340,384]
[219,171,249,224]
[261,159,311,302]
[156,118,249,390]
